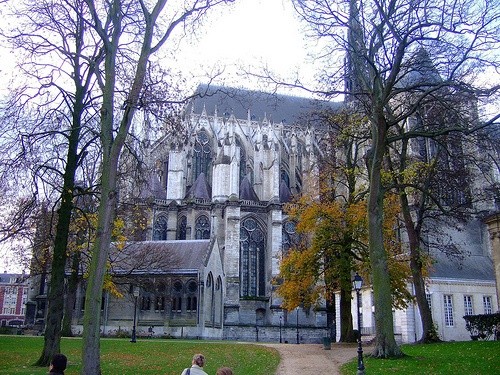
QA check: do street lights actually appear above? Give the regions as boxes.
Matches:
[279,316,282,343]
[130,290,139,343]
[296,305,300,344]
[352,271,366,375]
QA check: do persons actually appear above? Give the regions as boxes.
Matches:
[216,368,232,375]
[48,354,67,375]
[181,354,208,375]
